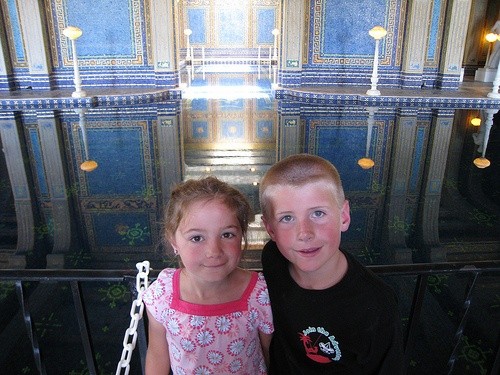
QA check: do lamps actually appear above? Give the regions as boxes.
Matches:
[271,65,278,90]
[184,28,192,60]
[62,26,87,98]
[366,25,387,97]
[358,107,378,170]
[272,29,281,61]
[74,109,98,172]
[186,64,192,96]
[473,109,499,168]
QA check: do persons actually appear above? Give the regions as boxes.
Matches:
[143,179,275,375]
[259,154,400,374]
